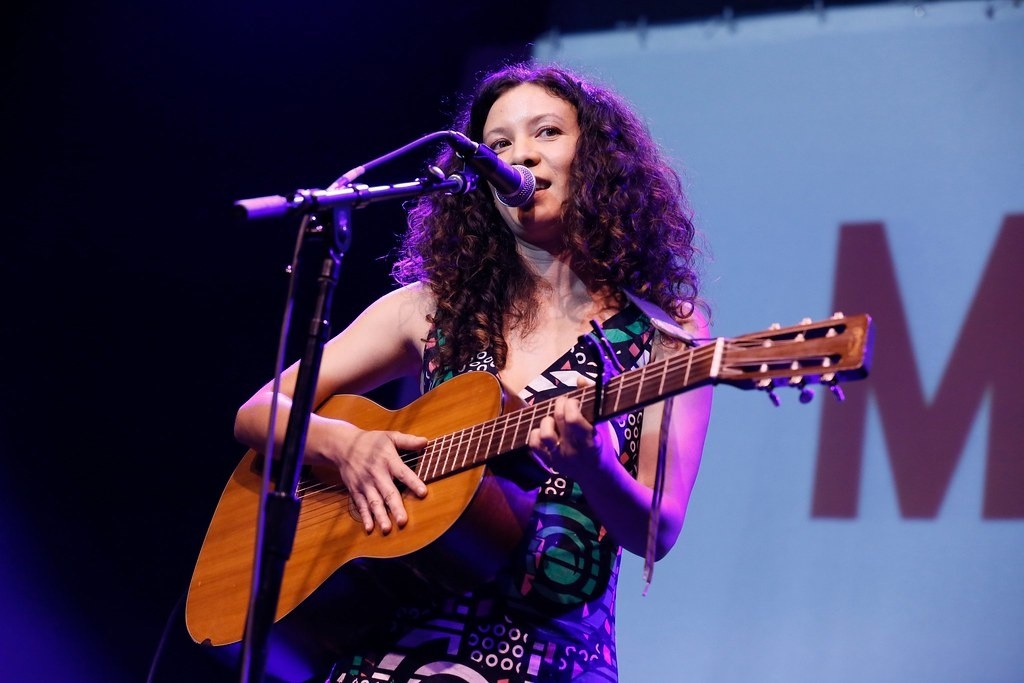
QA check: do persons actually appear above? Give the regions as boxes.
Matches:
[235,63,713,683]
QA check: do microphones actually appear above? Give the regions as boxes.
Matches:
[444,130,536,209]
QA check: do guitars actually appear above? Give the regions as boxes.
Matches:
[184,314,871,647]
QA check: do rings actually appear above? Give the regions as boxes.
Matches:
[551,441,560,453]
